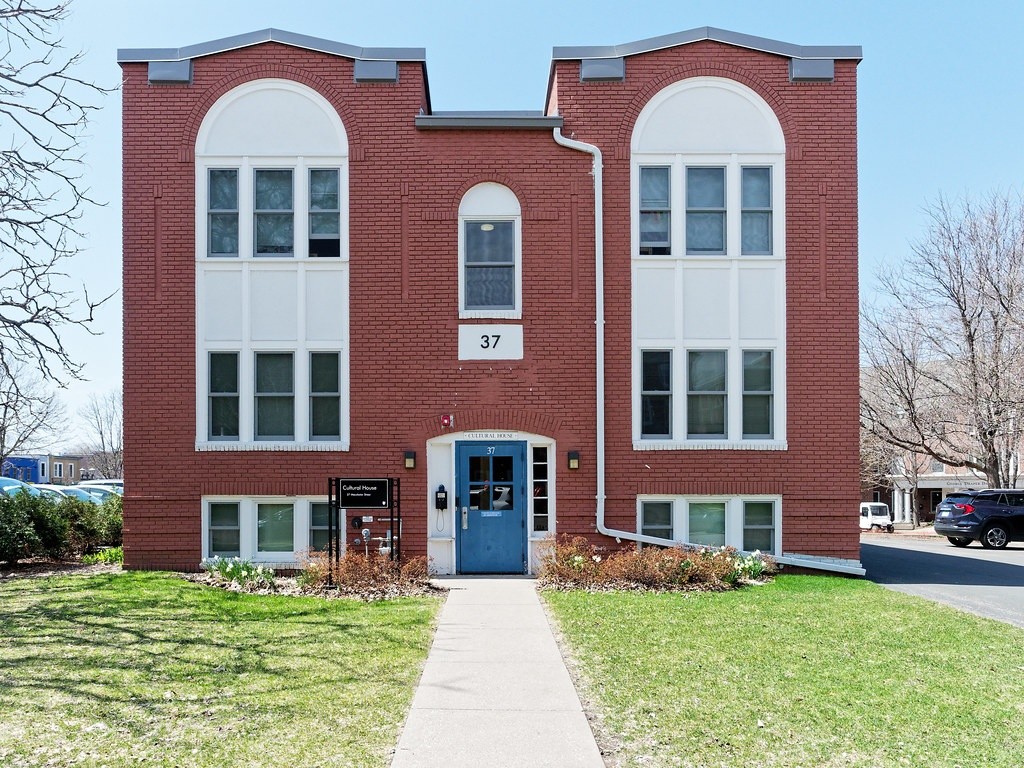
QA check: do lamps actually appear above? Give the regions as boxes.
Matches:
[405,450,417,469]
[569,451,579,469]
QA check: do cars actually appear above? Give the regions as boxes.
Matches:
[0,475,124,515]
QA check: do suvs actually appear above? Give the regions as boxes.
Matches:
[934,488,1024,550]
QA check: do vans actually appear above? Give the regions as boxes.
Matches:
[860,501,894,533]
